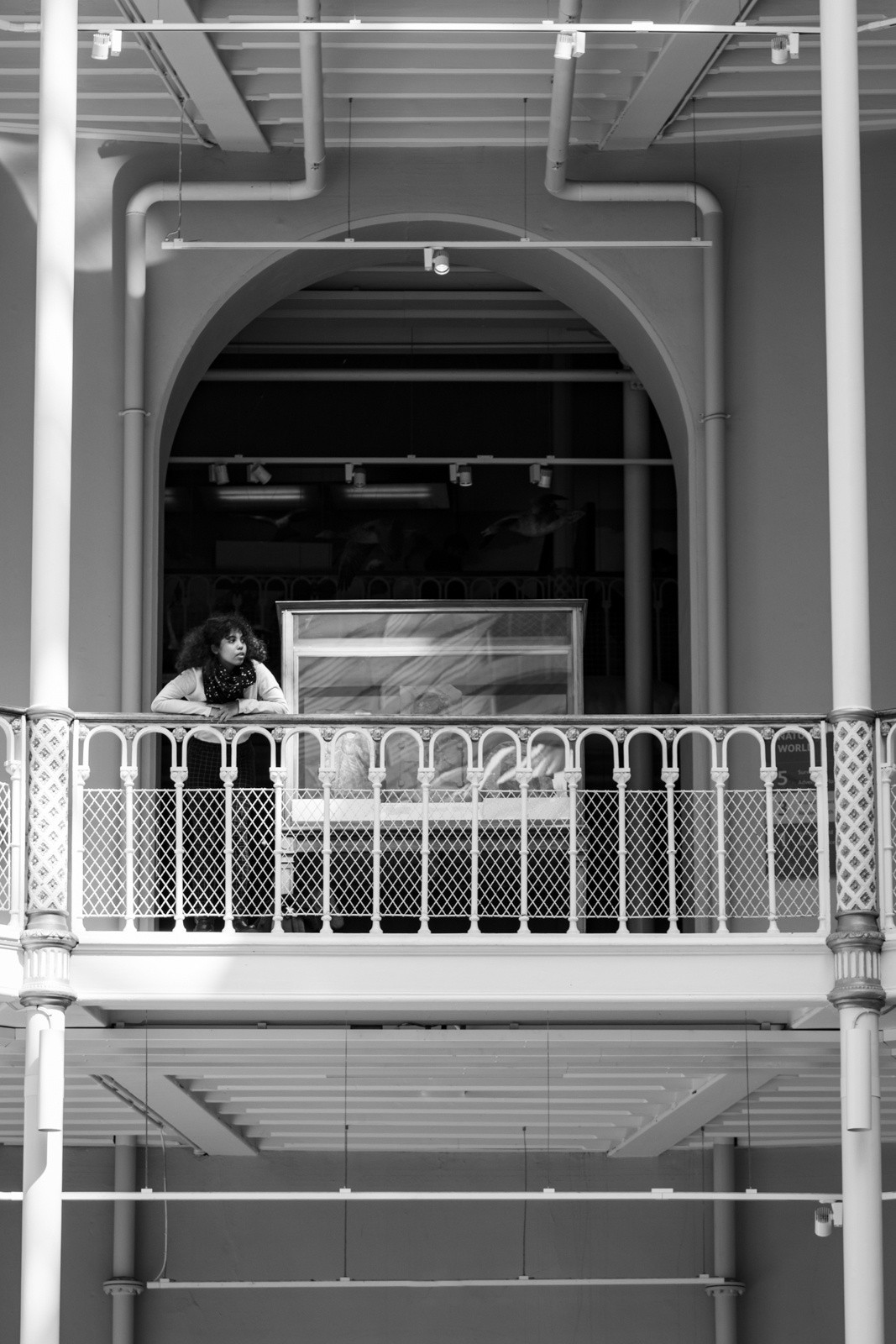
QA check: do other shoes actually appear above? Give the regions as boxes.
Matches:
[240,906,262,927]
[194,910,213,931]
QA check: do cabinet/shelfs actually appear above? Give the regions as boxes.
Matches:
[275,599,590,934]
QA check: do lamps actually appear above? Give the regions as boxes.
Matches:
[168,324,675,489]
[161,97,714,275]
[0,0,896,66]
[0,1008,896,1290]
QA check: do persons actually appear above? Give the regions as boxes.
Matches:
[152,616,288,932]
